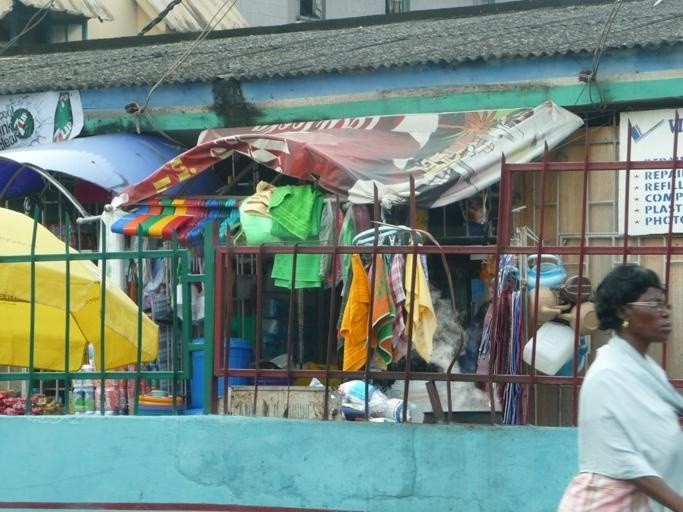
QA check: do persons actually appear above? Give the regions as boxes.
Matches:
[558,263,683,511]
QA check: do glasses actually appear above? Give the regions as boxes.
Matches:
[625,299,674,312]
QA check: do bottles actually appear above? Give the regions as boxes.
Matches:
[128,362,161,400]
[72,364,128,416]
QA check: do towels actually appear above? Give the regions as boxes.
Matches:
[372,245,397,372]
[271,183,326,291]
[389,253,406,349]
[338,255,352,352]
[339,251,370,373]
[407,251,439,364]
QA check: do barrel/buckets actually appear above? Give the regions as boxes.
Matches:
[522,319,575,376]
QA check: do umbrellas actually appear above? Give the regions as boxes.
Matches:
[0,206,158,371]
